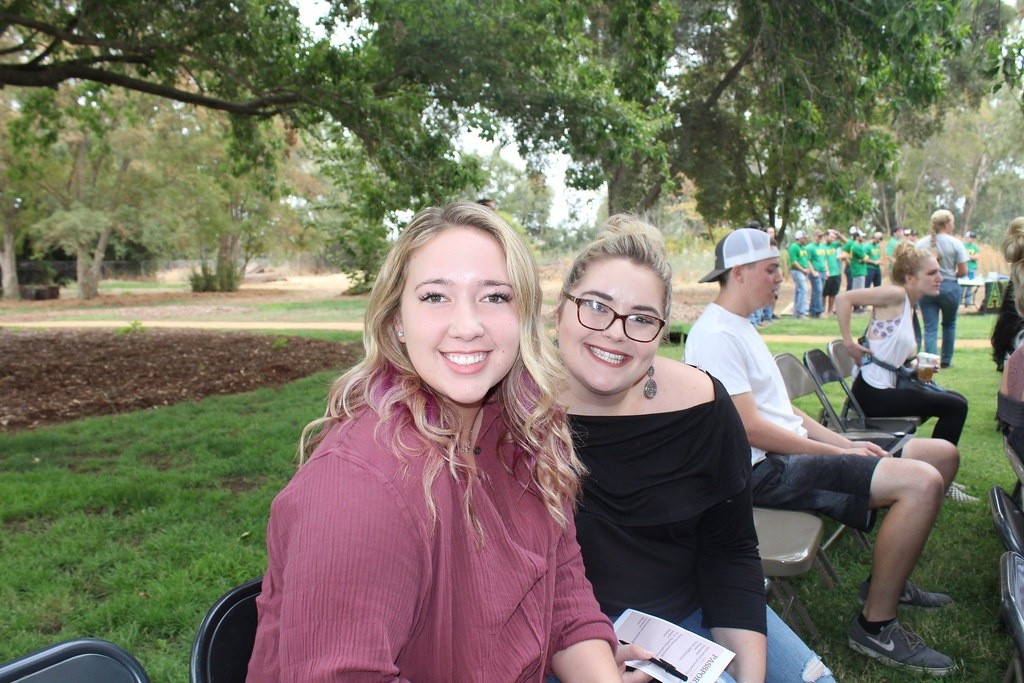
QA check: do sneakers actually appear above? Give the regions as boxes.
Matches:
[858,574,953,611]
[847,612,960,675]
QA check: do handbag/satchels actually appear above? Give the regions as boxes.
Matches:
[871,356,947,395]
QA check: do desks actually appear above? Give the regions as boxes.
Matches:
[957,278,986,311]
[980,279,1010,310]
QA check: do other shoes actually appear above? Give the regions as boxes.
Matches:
[945,481,980,505]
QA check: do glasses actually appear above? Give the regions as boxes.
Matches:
[562,289,666,342]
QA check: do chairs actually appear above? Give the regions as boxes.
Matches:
[776,353,898,450]
[0,637,151,683]
[988,487,1024,558]
[829,339,931,426]
[803,348,917,437]
[999,551,1024,654]
[754,506,825,634]
[189,576,264,683]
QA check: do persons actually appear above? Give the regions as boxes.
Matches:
[991,215,1024,460]
[684,228,960,675]
[246,198,625,683]
[913,209,971,368]
[727,220,979,331]
[835,241,982,503]
[542,214,840,683]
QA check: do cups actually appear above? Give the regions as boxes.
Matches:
[990,272,997,280]
[917,352,940,382]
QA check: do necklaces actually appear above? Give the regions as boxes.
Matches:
[460,403,483,454]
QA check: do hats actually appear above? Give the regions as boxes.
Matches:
[794,228,917,239]
[699,228,781,284]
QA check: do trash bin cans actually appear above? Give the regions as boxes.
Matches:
[986,275,1008,309]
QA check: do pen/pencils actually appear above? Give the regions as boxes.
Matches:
[619,637,690,681]
